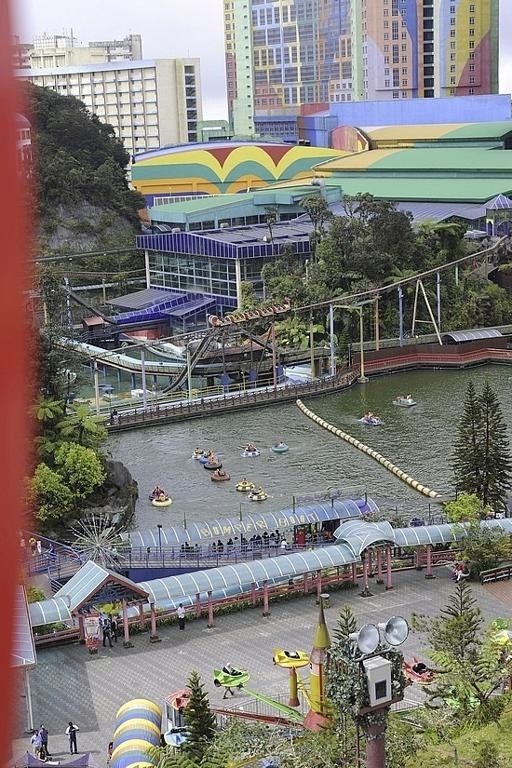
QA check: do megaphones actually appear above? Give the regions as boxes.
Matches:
[378,616,409,645]
[349,622,381,652]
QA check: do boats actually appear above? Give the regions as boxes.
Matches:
[396,397,417,406]
[361,417,382,426]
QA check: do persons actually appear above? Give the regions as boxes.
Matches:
[177,521,339,559]
[403,392,412,400]
[240,476,265,498]
[106,542,120,565]
[108,617,118,643]
[449,561,470,584]
[30,729,44,759]
[362,411,382,425]
[108,741,114,759]
[193,446,229,480]
[65,721,80,755]
[46,543,57,564]
[35,538,42,554]
[152,485,169,501]
[176,603,186,630]
[20,535,27,552]
[245,442,258,452]
[38,724,52,756]
[28,535,38,554]
[222,663,241,675]
[222,686,234,700]
[274,441,288,449]
[102,619,114,648]
[110,409,122,425]
